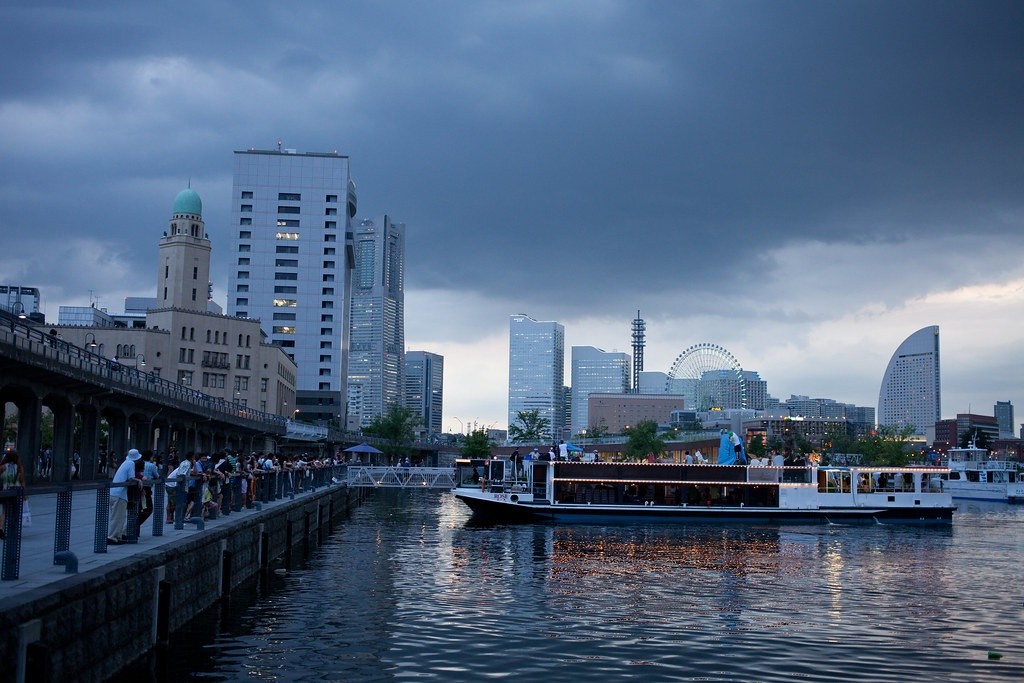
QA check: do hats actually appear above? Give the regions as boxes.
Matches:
[125,448,142,461]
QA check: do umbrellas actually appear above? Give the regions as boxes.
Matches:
[343,442,384,466]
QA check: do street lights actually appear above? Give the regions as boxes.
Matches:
[84,332,98,360]
[233,384,241,402]
[136,353,147,369]
[12,301,26,319]
[453,416,464,434]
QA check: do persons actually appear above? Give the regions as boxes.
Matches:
[839,473,927,493]
[390,455,426,482]
[624,484,778,507]
[685,451,694,464]
[111,356,119,372]
[0,448,361,545]
[750,452,806,483]
[509,439,599,479]
[729,431,742,460]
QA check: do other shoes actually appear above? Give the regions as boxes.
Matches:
[0,529,4,539]
[107,538,126,545]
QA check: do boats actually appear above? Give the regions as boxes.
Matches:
[927,430,1024,505]
[451,457,957,525]
